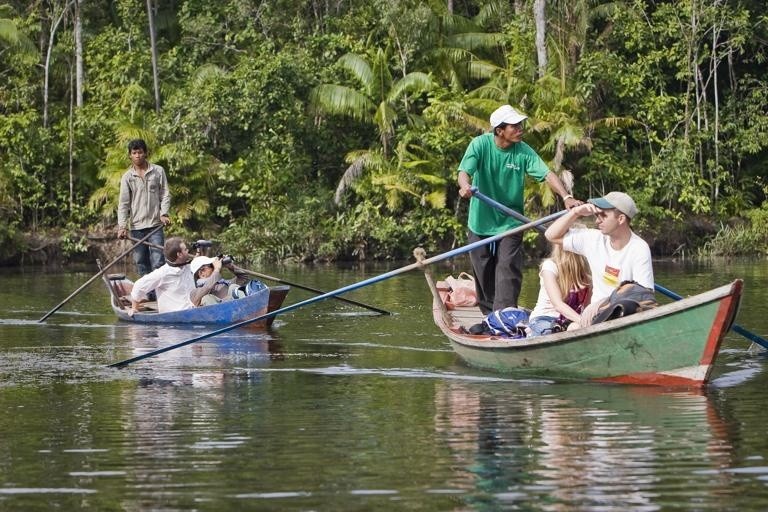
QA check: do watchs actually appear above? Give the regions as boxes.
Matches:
[160,213,169,217]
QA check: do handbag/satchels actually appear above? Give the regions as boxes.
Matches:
[485,307,530,339]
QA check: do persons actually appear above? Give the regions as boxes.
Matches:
[588,414,665,512]
[126,324,165,453]
[116,138,171,302]
[150,327,192,429]
[538,395,592,511]
[454,103,588,317]
[458,386,528,511]
[191,339,237,475]
[526,222,589,337]
[125,236,209,314]
[189,253,252,308]
[541,191,655,328]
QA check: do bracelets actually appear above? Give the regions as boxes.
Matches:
[573,206,583,217]
[561,194,574,201]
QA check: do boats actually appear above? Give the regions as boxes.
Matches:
[101,271,290,330]
[432,271,745,390]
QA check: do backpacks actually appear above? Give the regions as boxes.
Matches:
[232,279,267,299]
[592,280,656,325]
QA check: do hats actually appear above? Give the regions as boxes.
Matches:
[191,256,216,272]
[587,191,635,220]
[490,104,528,127]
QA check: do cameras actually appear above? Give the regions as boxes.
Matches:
[217,254,231,265]
